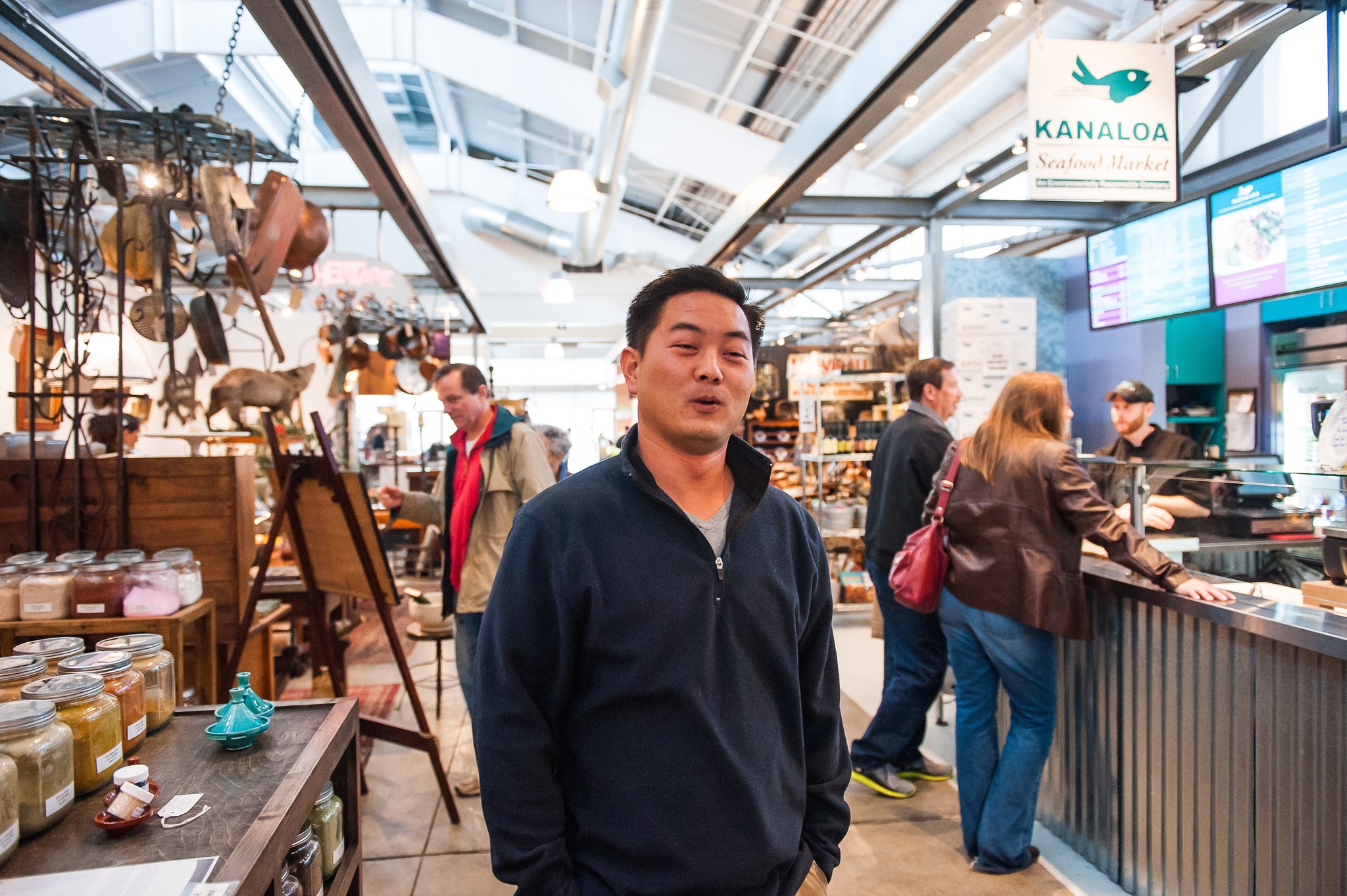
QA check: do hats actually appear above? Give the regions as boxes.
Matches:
[1104,381,1154,403]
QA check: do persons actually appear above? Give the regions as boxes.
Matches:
[849,358,962,797]
[888,373,1239,874]
[88,412,141,458]
[379,363,575,797]
[470,266,852,896]
[1086,381,1212,537]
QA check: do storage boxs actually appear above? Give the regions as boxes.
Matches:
[811,506,857,531]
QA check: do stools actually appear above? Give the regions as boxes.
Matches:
[407,622,457,719]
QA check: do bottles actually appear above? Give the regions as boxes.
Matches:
[205,671,275,750]
[822,419,891,454]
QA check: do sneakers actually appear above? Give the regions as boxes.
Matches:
[896,755,953,781]
[852,761,917,798]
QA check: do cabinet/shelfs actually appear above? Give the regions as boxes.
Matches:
[0,697,364,896]
[748,372,912,579]
[0,598,217,707]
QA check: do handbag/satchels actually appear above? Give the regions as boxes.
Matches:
[888,437,972,616]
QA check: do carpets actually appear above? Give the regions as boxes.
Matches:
[276,684,400,768]
[329,586,421,662]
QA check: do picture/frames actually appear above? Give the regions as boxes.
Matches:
[16,324,65,431]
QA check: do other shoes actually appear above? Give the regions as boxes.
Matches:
[1026,845,1040,863]
[454,774,480,796]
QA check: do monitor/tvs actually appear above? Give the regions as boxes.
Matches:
[1224,454,1295,496]
